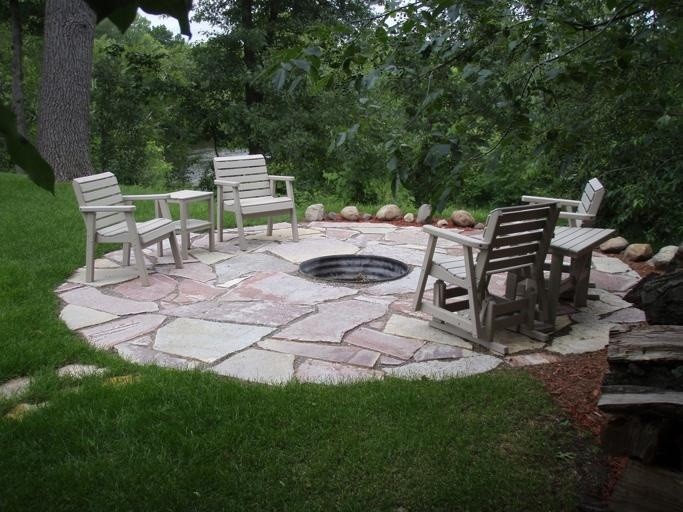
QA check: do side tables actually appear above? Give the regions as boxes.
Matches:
[155,188,217,257]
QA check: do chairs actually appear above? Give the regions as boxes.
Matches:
[412,175,604,356]
[212,153,301,251]
[73,170,184,286]
